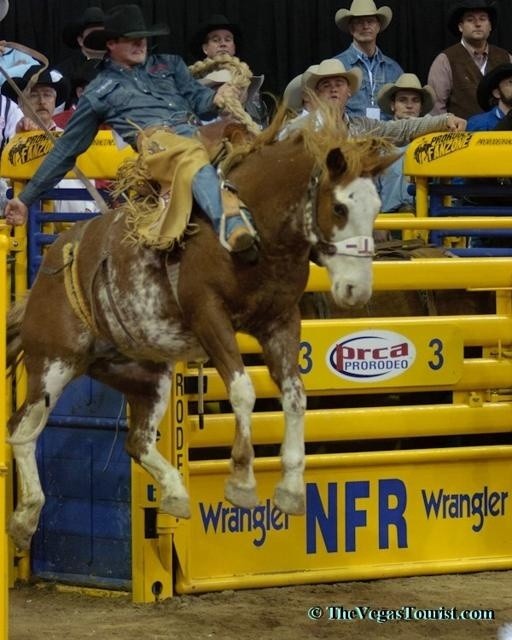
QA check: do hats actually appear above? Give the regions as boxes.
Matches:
[376,72,436,118]
[80,3,171,60]
[476,64,512,112]
[335,0,394,34]
[0,66,73,109]
[282,71,306,113]
[65,67,102,111]
[185,12,246,61]
[445,0,506,45]
[303,59,364,102]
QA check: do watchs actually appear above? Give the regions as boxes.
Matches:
[446,112,454,124]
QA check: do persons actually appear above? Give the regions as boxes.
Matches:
[52,68,130,211]
[53,6,108,115]
[281,58,467,147]
[188,15,271,132]
[278,64,319,141]
[332,0,404,122]
[362,73,438,243]
[3,3,260,265]
[450,62,512,248]
[428,0,512,121]
[0,64,101,236]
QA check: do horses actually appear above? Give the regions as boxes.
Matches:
[7,89,407,553]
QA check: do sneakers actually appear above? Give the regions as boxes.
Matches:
[225,226,260,264]
